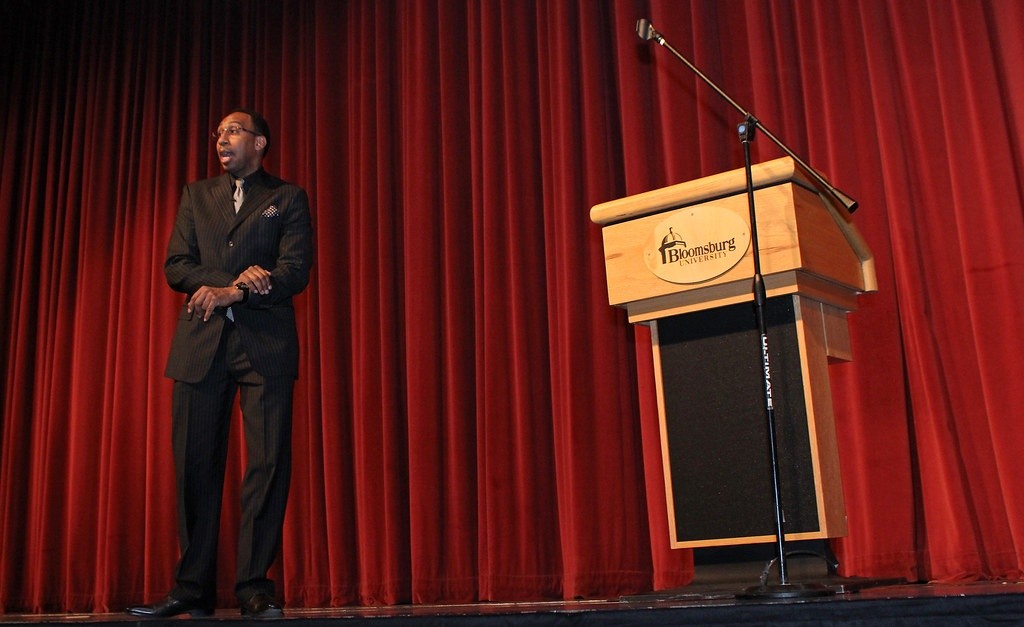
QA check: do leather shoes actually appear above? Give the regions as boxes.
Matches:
[126,595,216,617]
[240,592,283,617]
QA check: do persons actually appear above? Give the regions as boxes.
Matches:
[125,109,314,619]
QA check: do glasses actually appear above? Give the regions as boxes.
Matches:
[212,125,259,137]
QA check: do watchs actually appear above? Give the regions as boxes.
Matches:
[235,281,249,305]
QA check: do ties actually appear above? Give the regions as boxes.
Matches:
[232,178,245,214]
